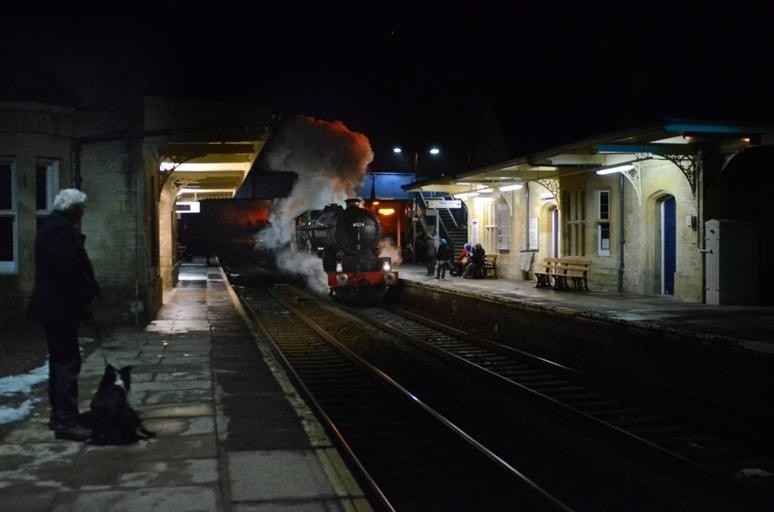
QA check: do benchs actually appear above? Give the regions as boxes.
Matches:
[454,252,497,279]
[532,258,590,292]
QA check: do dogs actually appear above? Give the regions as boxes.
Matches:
[83,363,157,446]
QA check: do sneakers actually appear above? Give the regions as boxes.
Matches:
[54,420,92,440]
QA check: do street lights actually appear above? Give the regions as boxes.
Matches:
[393,143,443,263]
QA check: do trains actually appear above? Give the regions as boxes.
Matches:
[257,202,405,309]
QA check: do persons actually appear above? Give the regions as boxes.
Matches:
[454,244,473,276]
[20,186,107,445]
[460,243,486,279]
[433,238,449,279]
[419,231,435,276]
